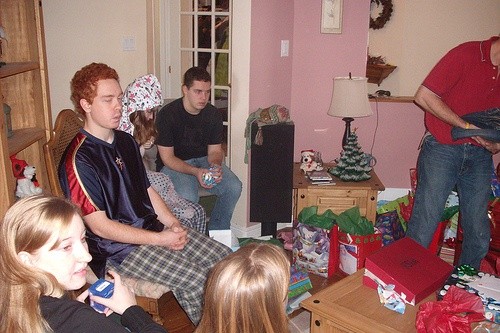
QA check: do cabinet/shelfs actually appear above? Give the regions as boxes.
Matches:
[0,0,54,226]
[291,161,389,229]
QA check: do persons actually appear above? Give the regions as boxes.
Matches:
[0,194,169,333]
[406,35,500,270]
[57,63,232,328]
[156,67,242,246]
[114,74,206,236]
[192,242,291,333]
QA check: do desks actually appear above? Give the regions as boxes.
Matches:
[298,263,500,333]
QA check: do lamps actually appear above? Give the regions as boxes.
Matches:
[327,72,373,164]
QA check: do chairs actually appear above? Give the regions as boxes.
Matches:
[42,108,179,327]
[147,98,217,201]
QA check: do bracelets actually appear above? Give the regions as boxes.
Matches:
[465,122,470,129]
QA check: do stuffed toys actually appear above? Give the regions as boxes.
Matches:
[300,149,323,174]
[9,155,43,198]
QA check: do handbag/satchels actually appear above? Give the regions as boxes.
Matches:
[291,218,340,278]
[338,226,382,278]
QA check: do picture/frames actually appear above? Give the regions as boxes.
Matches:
[321,0,343,33]
